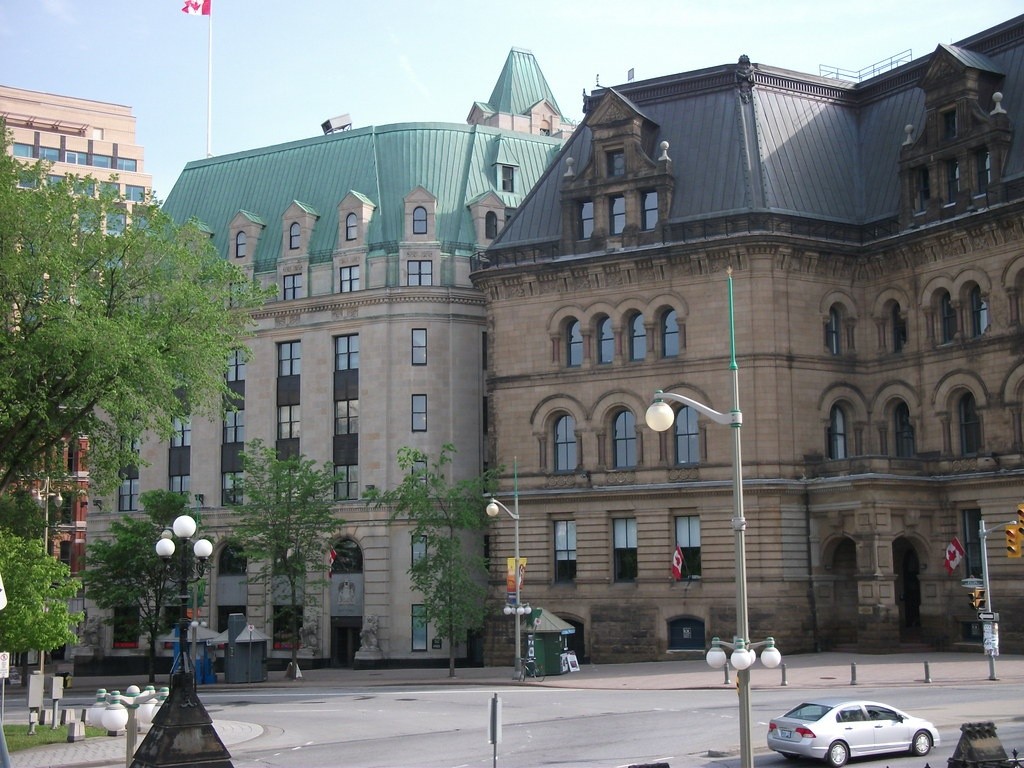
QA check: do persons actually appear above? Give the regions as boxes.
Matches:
[519,564,525,586]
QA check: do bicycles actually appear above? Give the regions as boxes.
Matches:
[515,656,547,682]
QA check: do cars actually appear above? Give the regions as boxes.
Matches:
[764,696,944,768]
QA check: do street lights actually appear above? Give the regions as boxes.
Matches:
[87,683,170,768]
[155,513,213,672]
[30,476,65,673]
[190,490,209,687]
[486,454,533,680]
[645,263,784,766]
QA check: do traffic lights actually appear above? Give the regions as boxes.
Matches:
[966,592,977,610]
[975,588,987,609]
[1016,501,1024,542]
[1003,523,1021,558]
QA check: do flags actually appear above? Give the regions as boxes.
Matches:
[182,0,211,16]
[328,549,337,578]
[673,545,684,581]
[943,538,966,576]
